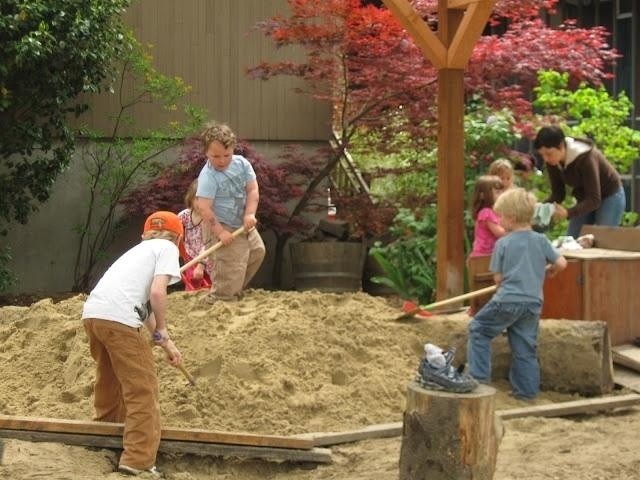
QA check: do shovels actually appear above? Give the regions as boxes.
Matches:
[395,265,552,320]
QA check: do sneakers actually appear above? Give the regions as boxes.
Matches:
[415,346,479,393]
[119,460,163,478]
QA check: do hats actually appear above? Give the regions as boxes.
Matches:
[143,210,186,239]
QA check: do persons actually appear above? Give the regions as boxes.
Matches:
[490,159,513,190]
[466,175,510,317]
[179,174,214,291]
[463,187,567,402]
[195,126,266,302]
[80,211,182,479]
[533,125,627,240]
